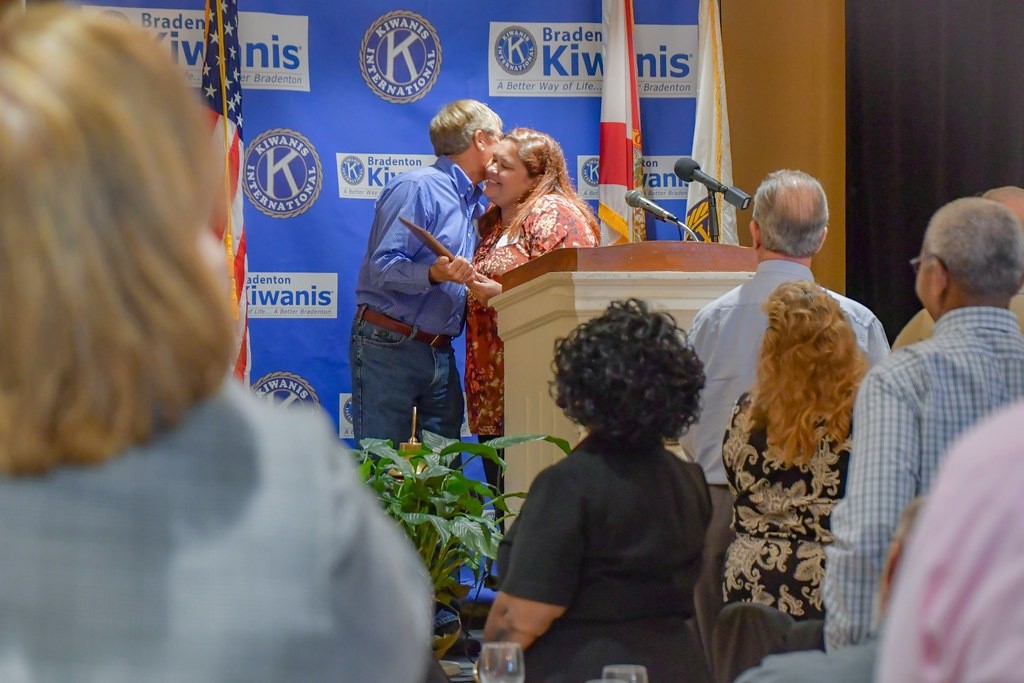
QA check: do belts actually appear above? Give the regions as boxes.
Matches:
[355,305,453,349]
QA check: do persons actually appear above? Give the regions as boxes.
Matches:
[677,168,891,670]
[822,198,1024,653]
[891,184,1024,353]
[719,279,871,618]
[0,5,436,683]
[735,497,933,683]
[465,126,602,528]
[349,99,504,654]
[872,397,1024,683]
[474,297,714,683]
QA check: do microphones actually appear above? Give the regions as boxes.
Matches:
[673,157,752,211]
[624,189,679,221]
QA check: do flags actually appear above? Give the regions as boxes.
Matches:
[683,0,741,245]
[200,0,252,390]
[598,0,648,247]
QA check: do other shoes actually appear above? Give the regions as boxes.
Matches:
[462,603,489,618]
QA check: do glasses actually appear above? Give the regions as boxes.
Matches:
[910,253,947,274]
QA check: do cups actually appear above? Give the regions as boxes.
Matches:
[586,679,629,683]
[602,664,649,683]
[480,642,525,683]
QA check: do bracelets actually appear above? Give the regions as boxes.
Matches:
[472,660,479,682]
